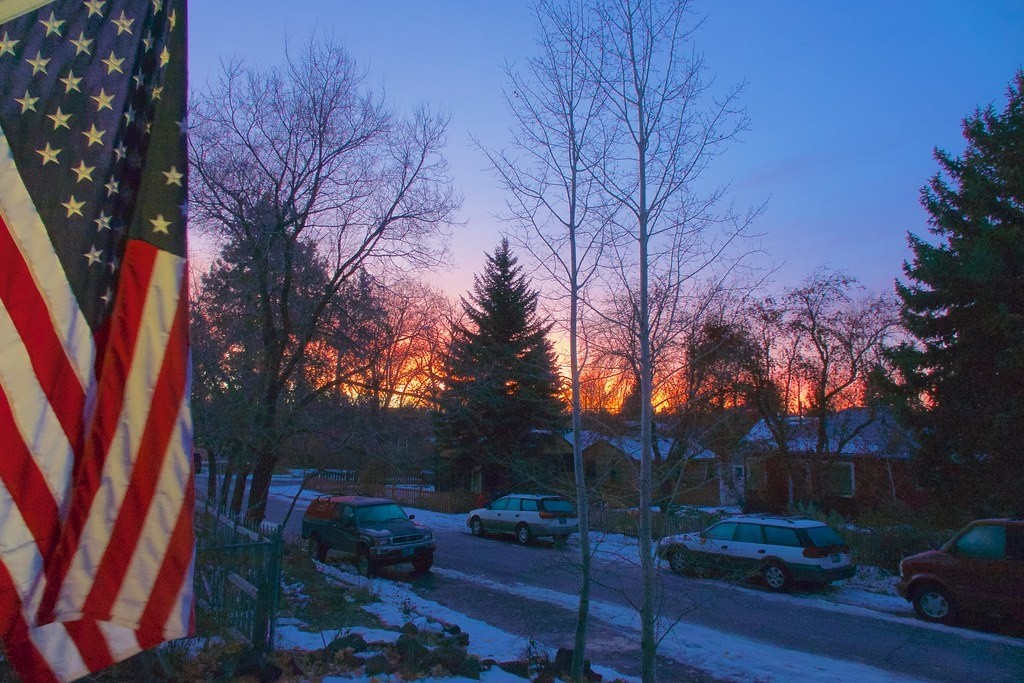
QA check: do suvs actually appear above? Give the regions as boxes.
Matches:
[657,515,857,594]
[301,495,436,578]
[467,492,588,546]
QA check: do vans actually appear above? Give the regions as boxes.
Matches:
[894,516,1024,640]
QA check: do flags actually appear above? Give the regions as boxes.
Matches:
[0,0,195,683]
